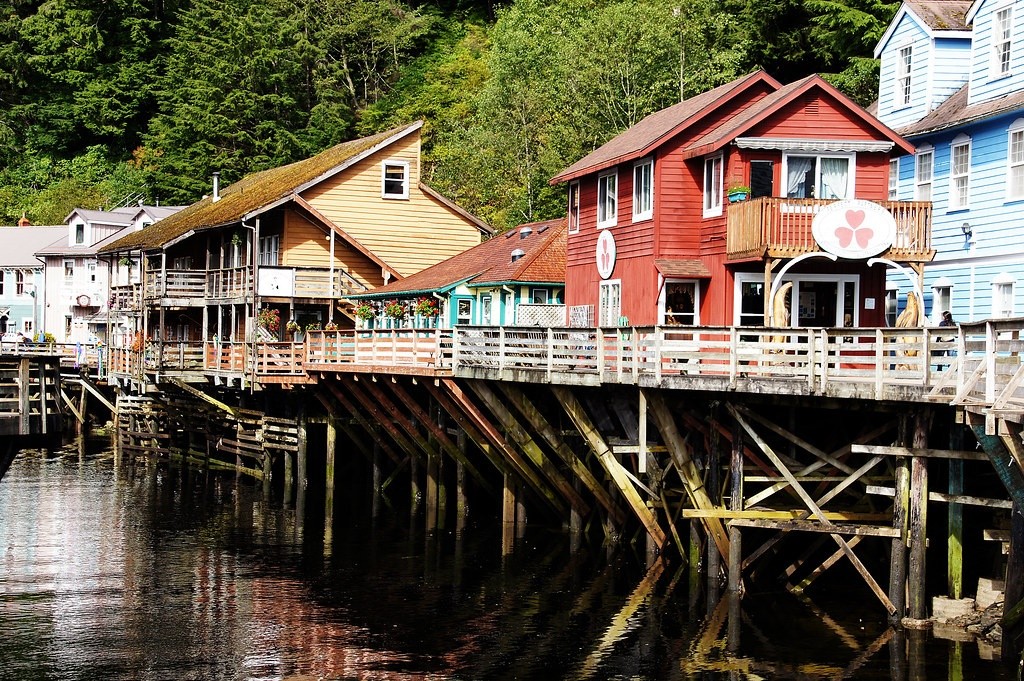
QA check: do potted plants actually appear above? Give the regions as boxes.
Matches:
[286,320,301,340]
[726,184,752,203]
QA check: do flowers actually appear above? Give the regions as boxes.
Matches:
[415,296,439,317]
[354,301,375,320]
[383,298,405,319]
[258,307,280,333]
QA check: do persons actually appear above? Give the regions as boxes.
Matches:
[936,311,958,371]
[38,330,46,352]
[22,337,34,352]
